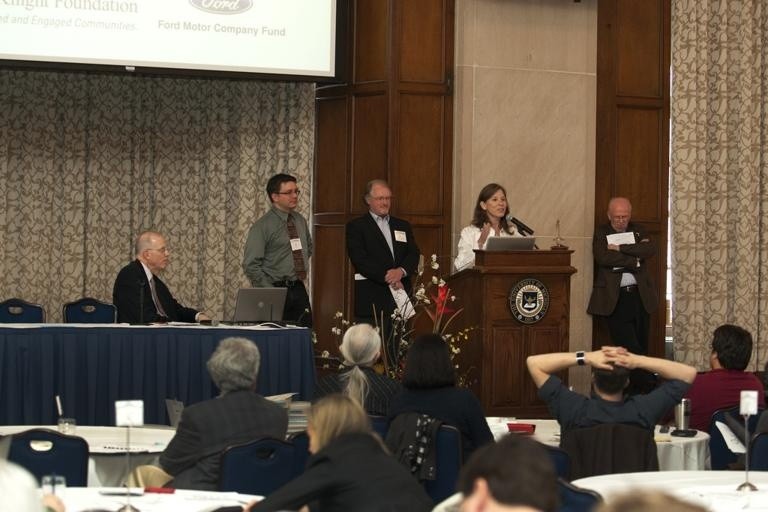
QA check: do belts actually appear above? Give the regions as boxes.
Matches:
[622,285,638,292]
[275,280,300,287]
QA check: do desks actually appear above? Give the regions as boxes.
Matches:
[1,324,313,427]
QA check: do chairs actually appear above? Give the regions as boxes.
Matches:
[0,298,46,324]
[0,400,766,511]
[63,296,118,323]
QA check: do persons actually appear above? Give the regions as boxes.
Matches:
[454,184,527,272]
[113,232,211,324]
[161,323,764,511]
[242,173,312,328]
[587,197,659,394]
[344,180,420,376]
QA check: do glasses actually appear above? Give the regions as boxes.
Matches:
[275,191,300,196]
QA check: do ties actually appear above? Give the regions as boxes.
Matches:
[151,277,165,316]
[287,213,306,281]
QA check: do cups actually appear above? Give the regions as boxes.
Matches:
[674,398,692,430]
[43,476,66,501]
[58,418,75,434]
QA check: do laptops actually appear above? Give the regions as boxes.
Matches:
[486,236,536,250]
[219,287,287,326]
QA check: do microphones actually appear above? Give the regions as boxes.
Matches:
[506,215,534,235]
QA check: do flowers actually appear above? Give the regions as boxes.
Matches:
[310,252,482,393]
